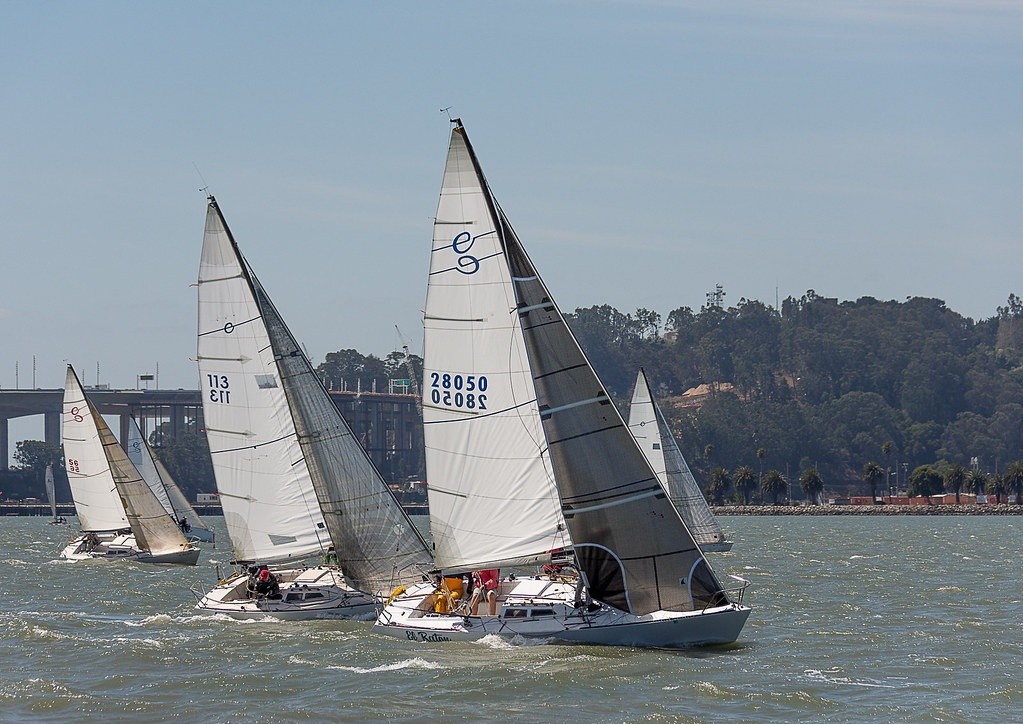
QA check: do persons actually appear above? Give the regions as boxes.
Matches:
[471,569,499,615]
[179,515,190,534]
[245,565,283,600]
[544,547,564,574]
[434,572,473,614]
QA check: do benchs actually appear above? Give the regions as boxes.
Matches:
[461,580,521,602]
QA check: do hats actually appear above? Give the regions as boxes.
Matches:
[259,570,269,582]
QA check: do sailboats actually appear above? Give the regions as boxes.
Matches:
[127,413,216,544]
[627,367,734,552]
[189,186,438,621]
[44,465,67,524]
[59,363,202,566]
[370,105,753,650]
